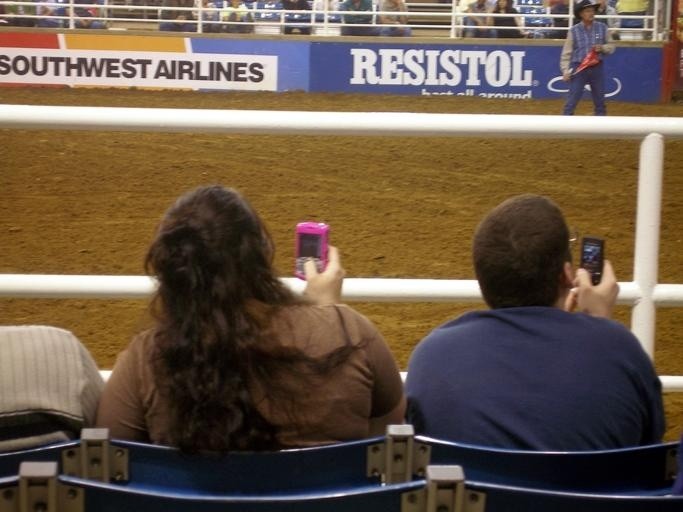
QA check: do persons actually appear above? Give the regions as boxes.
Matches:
[560,0,617,115]
[91,182,404,460]
[1,0,108,30]
[157,0,255,34]
[0,325,106,452]
[281,0,416,37]
[405,191,667,450]
[460,0,650,40]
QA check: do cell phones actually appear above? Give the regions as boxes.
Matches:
[294,222,329,281]
[580,236,604,285]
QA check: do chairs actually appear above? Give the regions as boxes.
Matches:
[0,425,683,512]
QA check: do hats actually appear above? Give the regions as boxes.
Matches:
[574,0,600,19]
